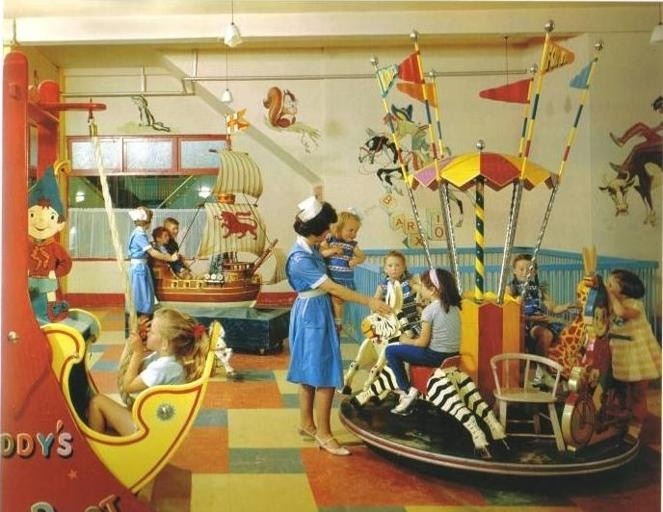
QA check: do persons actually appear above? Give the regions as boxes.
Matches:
[386,269,461,414]
[602,270,662,446]
[371,250,424,334]
[123,206,181,338]
[151,227,171,262]
[504,253,576,388]
[162,216,192,279]
[284,194,392,459]
[88,306,208,442]
[319,211,367,339]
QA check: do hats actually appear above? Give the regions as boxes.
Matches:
[298,197,322,223]
[128,209,146,222]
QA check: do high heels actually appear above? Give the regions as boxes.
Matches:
[296,425,319,441]
[315,435,351,455]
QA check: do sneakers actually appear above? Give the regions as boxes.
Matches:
[624,432,638,444]
[335,324,343,333]
[391,388,418,416]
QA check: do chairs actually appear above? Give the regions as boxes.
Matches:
[489,350,571,452]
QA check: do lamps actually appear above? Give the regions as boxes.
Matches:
[223,1,242,46]
